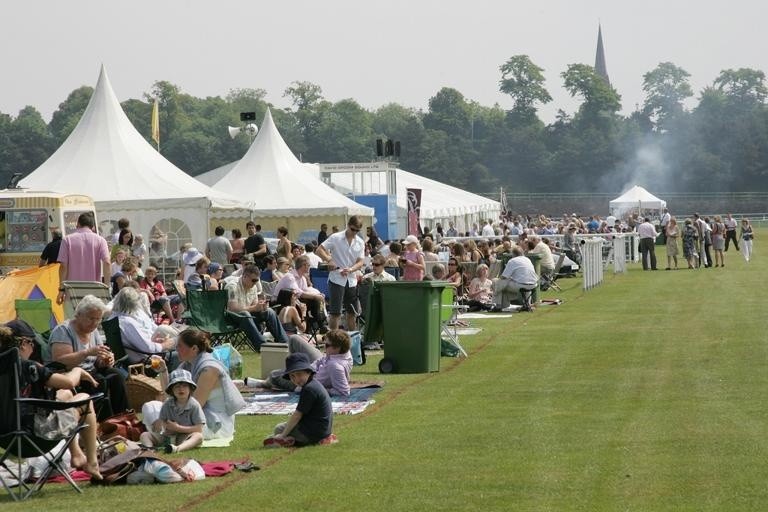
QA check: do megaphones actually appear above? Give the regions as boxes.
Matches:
[229,126,241,139]
[240,112,255,121]
[246,123,258,136]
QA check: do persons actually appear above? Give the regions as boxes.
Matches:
[555,213,641,263]
[142,330,245,439]
[666,218,679,270]
[38,211,112,305]
[176,221,338,352]
[661,208,671,245]
[725,213,740,251]
[244,330,353,397]
[48,295,129,424]
[741,219,752,260]
[105,217,183,369]
[452,214,555,313]
[141,369,206,453]
[363,222,461,288]
[638,218,658,270]
[263,353,336,448]
[2,319,103,481]
[681,212,726,269]
[316,215,364,332]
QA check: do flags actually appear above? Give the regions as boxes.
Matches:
[150,95,160,143]
[406,189,424,237]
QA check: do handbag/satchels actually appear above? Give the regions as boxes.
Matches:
[95,436,143,473]
[95,408,147,442]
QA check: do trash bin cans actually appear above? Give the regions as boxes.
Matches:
[363,278,449,374]
[503,253,543,305]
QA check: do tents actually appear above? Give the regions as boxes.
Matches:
[16,63,214,288]
[207,107,375,244]
[324,165,504,235]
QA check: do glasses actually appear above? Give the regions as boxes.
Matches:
[245,275,260,283]
[371,261,383,267]
[349,226,361,233]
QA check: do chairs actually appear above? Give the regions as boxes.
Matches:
[385,266,400,281]
[0,281,167,501]
[440,289,469,358]
[171,261,364,353]
[425,252,567,312]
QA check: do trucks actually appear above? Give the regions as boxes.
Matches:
[0,190,100,278]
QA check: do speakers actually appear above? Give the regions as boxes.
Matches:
[376,139,391,156]
[390,139,401,156]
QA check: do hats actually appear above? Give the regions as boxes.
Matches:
[283,352,318,378]
[5,318,36,338]
[183,245,204,265]
[402,235,419,245]
[164,368,197,395]
